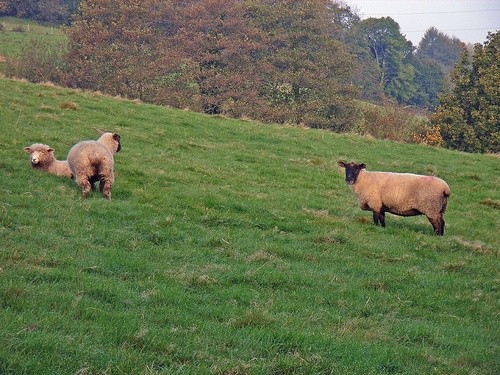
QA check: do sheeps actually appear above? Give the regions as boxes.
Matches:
[23,129,121,200]
[337,161,451,237]
